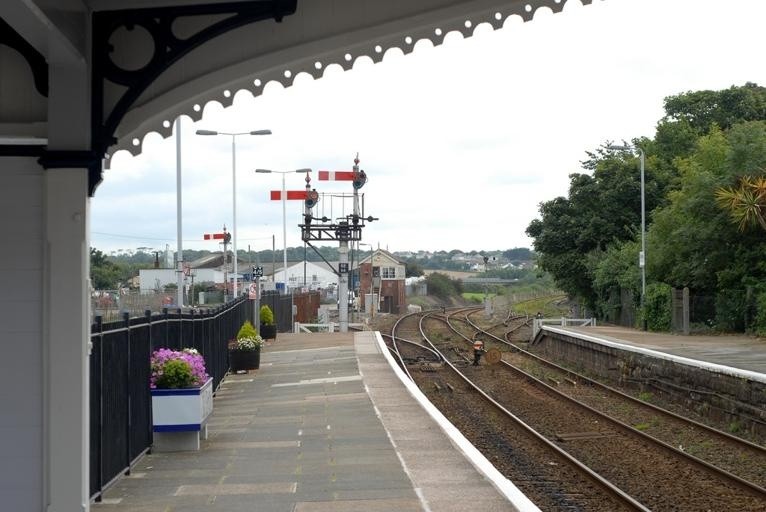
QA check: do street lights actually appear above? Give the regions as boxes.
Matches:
[196,129,272,301]
[255,169,313,293]
[614,146,645,297]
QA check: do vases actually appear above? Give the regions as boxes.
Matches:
[230,352,249,369]
[151,377,213,432]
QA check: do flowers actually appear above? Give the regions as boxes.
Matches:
[227,335,265,353]
[149,347,210,389]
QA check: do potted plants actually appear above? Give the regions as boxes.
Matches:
[260,305,276,339]
[237,319,260,370]
